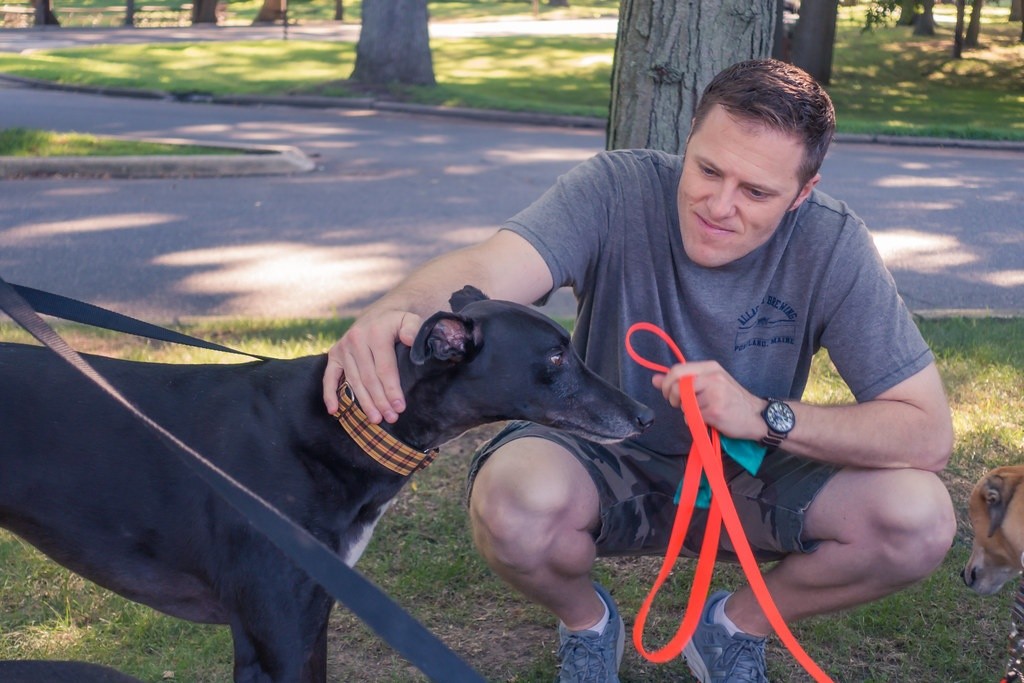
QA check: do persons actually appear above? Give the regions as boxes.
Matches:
[322,58,957,683]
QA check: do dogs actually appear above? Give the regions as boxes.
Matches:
[959,465,1023,596]
[0,284,657,683]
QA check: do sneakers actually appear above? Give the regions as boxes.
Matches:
[556,582,625,683]
[680,590,770,683]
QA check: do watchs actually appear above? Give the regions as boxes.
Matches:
[757,396,795,451]
